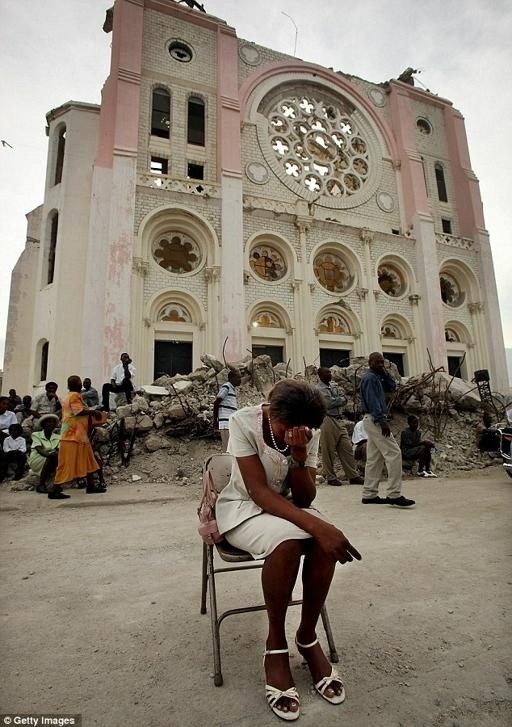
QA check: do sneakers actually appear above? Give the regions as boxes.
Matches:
[327,480,342,486]
[362,497,385,504]
[384,497,415,510]
[416,471,432,478]
[350,477,364,485]
[426,471,438,478]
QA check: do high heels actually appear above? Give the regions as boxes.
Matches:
[294,629,345,704]
[263,637,300,721]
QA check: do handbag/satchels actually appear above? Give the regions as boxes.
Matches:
[198,471,223,545]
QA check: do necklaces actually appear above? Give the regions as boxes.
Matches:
[266,414,290,452]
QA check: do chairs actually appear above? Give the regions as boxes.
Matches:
[200,453,339,686]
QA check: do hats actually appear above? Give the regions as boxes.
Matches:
[38,414,59,425]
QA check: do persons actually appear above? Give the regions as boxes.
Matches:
[212,369,241,453]
[352,418,367,460]
[215,378,362,720]
[401,415,437,478]
[0,353,134,499]
[316,366,364,486]
[360,352,415,506]
